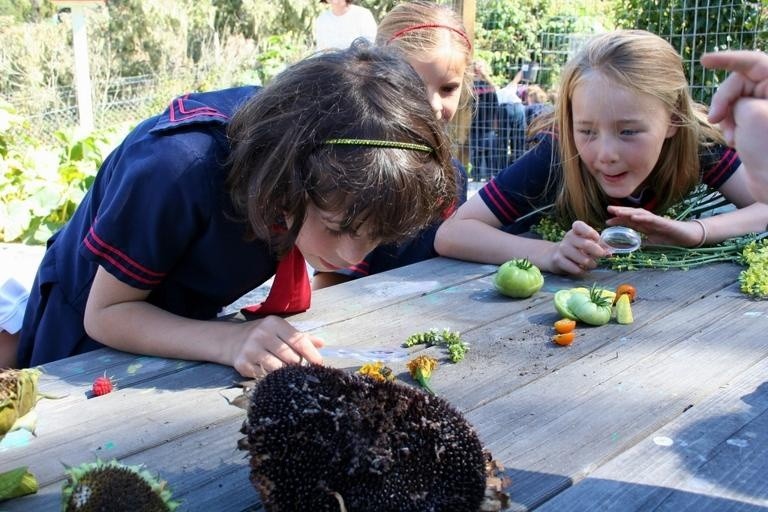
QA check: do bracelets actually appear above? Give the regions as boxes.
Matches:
[687,218,708,249]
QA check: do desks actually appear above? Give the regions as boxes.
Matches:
[0,223,768,507]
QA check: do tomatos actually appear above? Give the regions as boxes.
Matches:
[617,285,636,300]
[554,288,611,325]
[554,319,576,345]
[494,259,544,298]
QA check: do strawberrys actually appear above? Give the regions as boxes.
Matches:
[92,377,113,396]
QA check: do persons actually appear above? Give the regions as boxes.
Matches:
[433,29,768,277]
[461,58,562,186]
[310,1,380,61]
[696,49,768,205]
[311,0,476,292]
[16,37,454,383]
